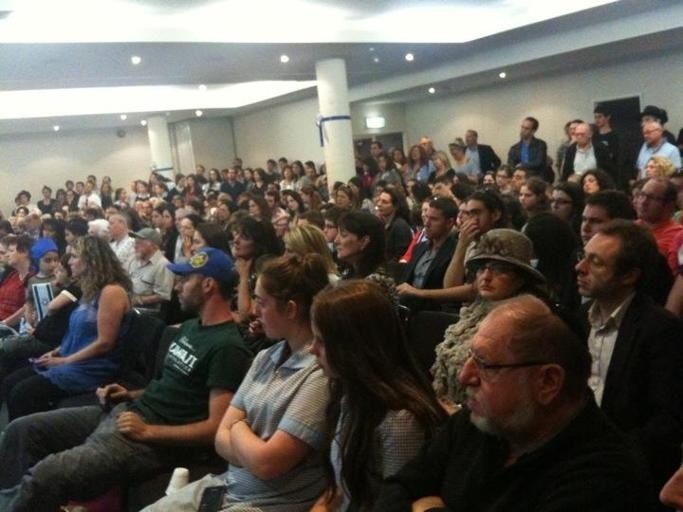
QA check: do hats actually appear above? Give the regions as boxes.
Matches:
[128,227,163,249]
[639,105,668,123]
[448,137,467,153]
[30,238,59,262]
[165,247,240,288]
[466,229,545,284]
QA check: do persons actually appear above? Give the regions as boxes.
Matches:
[0,104,683,511]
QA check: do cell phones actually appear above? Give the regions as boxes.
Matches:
[198,486,224,512]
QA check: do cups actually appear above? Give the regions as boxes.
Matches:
[165,466,190,497]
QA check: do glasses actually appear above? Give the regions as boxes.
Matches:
[638,190,667,204]
[465,345,553,383]
[321,222,337,230]
[548,196,573,205]
[575,249,627,273]
